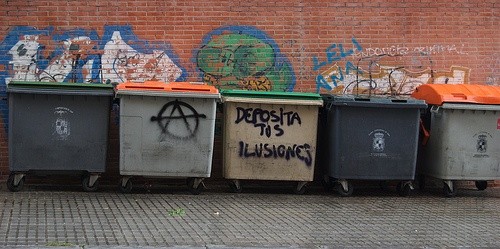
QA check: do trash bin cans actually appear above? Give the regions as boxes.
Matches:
[327,96,428,196]
[411,83,500,196]
[117,81,220,193]
[7,80,113,192]
[222,89,323,193]
